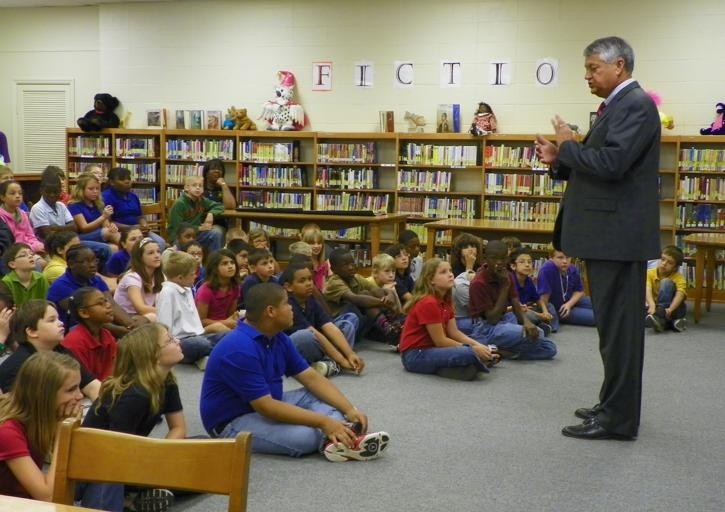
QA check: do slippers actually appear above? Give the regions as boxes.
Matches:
[486,351,504,368]
[437,363,478,381]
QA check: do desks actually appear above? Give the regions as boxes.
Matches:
[0,492,112,512]
[680,230,724,325]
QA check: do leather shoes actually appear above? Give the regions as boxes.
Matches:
[561,406,636,442]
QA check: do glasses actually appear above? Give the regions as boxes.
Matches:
[79,299,108,309]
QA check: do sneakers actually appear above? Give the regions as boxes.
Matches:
[323,428,391,464]
[647,306,687,334]
[125,485,175,512]
[196,355,210,371]
[385,324,405,352]
[314,359,342,377]
[538,322,552,337]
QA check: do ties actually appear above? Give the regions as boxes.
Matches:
[597,101,608,120]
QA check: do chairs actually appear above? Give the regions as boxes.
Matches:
[43,414,253,512]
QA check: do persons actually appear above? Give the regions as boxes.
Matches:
[700,101,725,135]
[644,245,691,333]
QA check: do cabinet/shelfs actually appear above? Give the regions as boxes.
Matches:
[115,127,164,240]
[677,134,725,302]
[66,127,114,194]
[238,130,570,261]
[164,128,237,217]
[658,135,677,250]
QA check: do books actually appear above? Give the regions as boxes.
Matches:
[675,148,725,291]
